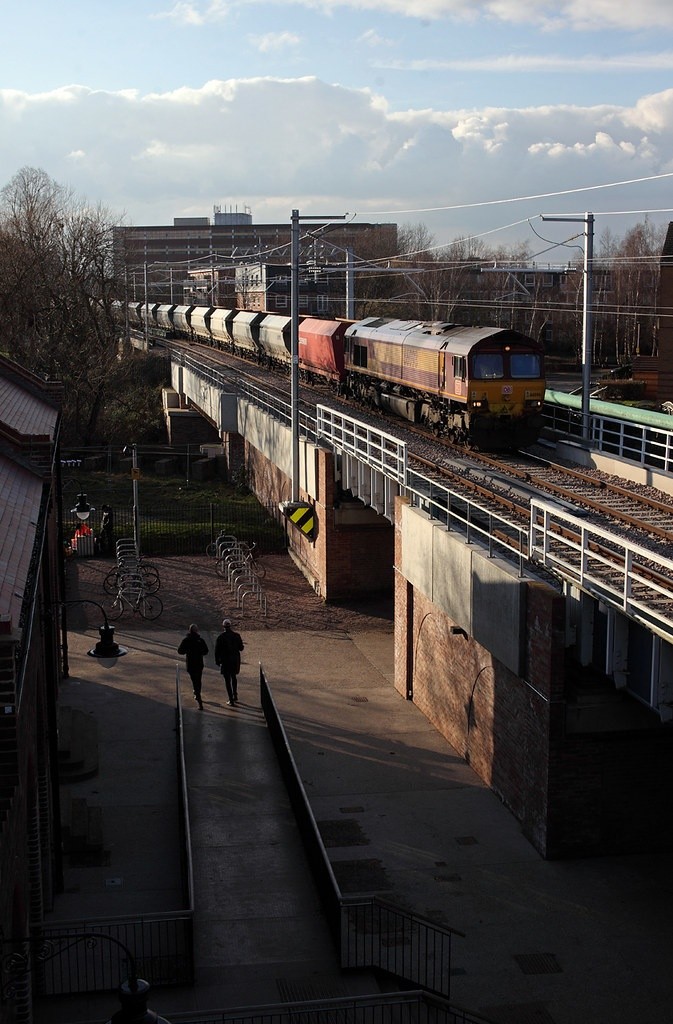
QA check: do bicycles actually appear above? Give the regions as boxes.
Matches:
[103,554,164,622]
[205,529,266,581]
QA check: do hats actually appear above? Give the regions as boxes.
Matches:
[189,623,198,632]
[222,619,230,626]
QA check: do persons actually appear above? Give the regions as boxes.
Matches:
[100,505,113,558]
[178,623,209,711]
[215,618,244,707]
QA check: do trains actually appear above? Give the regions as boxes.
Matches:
[52,295,547,455]
